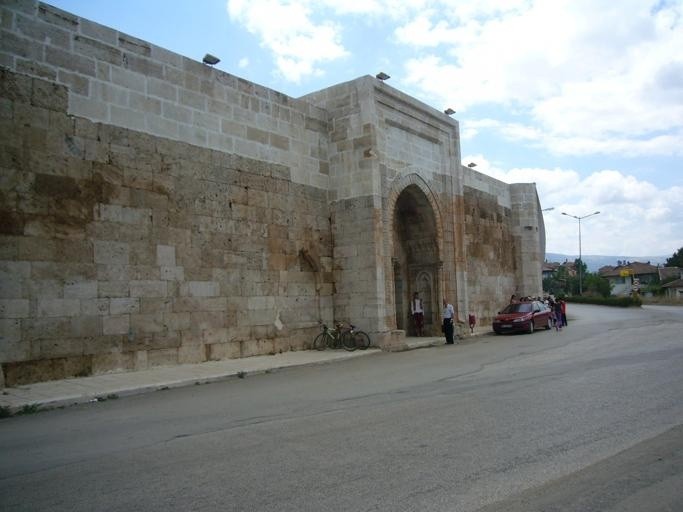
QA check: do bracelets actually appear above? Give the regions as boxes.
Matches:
[451,319,453,320]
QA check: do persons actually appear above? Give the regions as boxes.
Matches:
[442,297,455,344]
[509,293,568,332]
[410,292,425,337]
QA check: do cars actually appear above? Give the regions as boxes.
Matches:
[492,301,554,334]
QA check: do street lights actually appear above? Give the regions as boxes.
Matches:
[561,211,601,295]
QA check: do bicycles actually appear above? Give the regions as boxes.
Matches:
[311,318,371,352]
